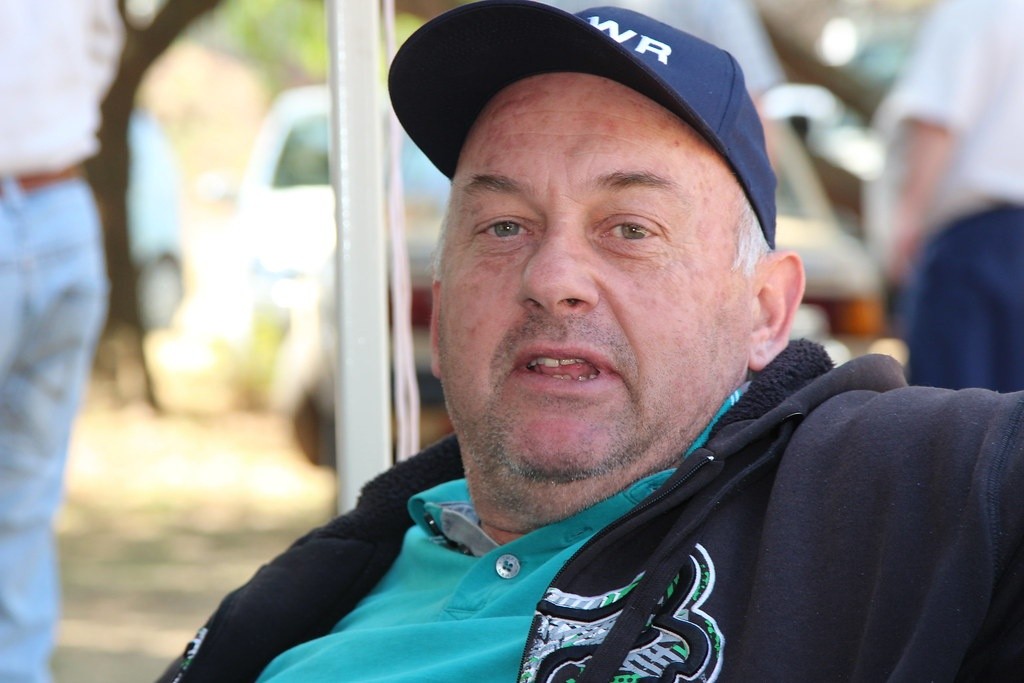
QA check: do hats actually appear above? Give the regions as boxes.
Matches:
[387,0,776,248]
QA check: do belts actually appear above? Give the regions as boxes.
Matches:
[0,167,76,202]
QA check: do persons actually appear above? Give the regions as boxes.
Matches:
[156,1,1024,683]
[0,0,128,683]
[870,1,1022,392]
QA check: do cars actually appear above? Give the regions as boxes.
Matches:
[234,84,890,466]
